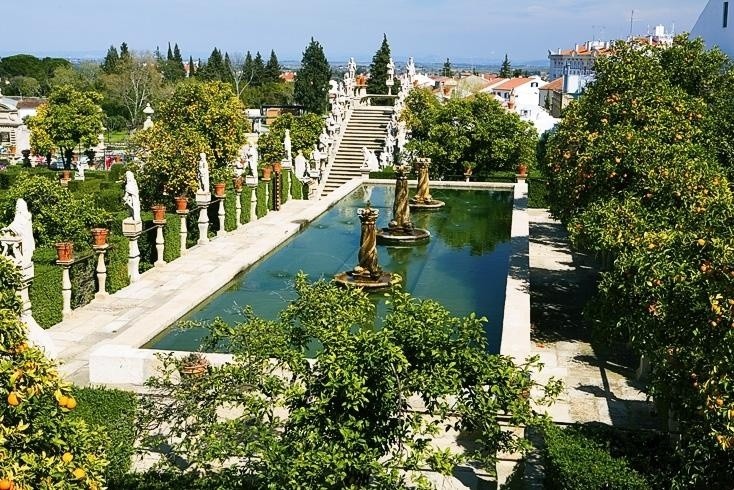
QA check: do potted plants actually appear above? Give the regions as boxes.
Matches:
[147,171,230,220]
[256,142,289,180]
[45,208,116,261]
[516,157,531,175]
[178,353,209,380]
[463,160,476,173]
